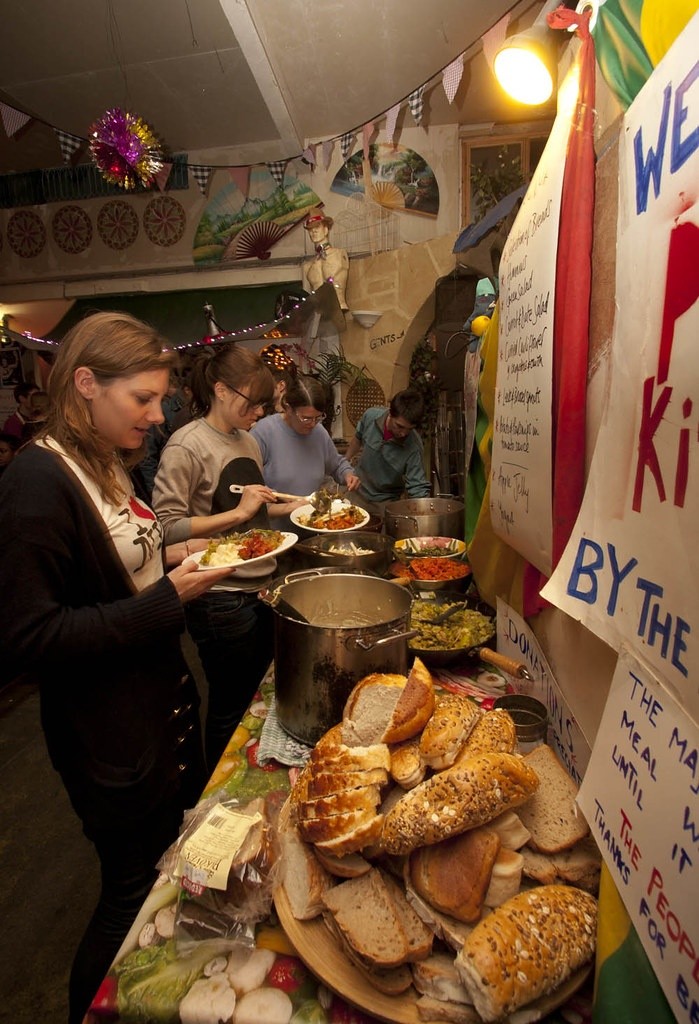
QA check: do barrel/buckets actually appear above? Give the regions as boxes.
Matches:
[493,694,549,755]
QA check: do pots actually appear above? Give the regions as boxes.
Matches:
[356,514,383,532]
[296,533,395,567]
[271,570,418,747]
[276,565,411,586]
[410,592,534,683]
[384,493,466,540]
[388,558,473,593]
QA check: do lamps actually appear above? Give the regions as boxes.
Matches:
[493,0,568,109]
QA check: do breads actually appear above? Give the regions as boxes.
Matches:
[169,655,599,1023]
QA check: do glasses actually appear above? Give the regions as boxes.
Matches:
[226,384,268,409]
[291,406,326,424]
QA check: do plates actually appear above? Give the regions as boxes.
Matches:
[182,531,299,577]
[392,536,466,559]
[273,867,592,1022]
[290,502,370,533]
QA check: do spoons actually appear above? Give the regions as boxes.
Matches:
[419,602,466,626]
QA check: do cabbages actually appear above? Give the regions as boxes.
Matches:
[113,942,216,1024]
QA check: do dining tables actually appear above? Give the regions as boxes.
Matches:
[83,642,680,1023]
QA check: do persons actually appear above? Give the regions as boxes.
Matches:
[344,387,433,536]
[248,373,362,580]
[20,391,55,442]
[1,309,238,1024]
[0,433,24,473]
[2,382,41,435]
[149,344,310,774]
[299,209,350,312]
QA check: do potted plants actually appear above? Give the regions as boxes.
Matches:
[294,343,372,438]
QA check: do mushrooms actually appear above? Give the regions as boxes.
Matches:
[139,903,180,952]
